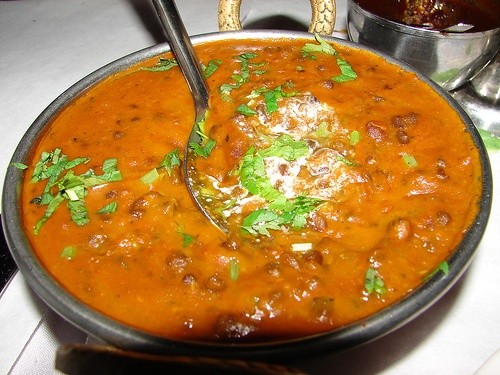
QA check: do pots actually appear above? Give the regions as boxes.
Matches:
[0,1,495,375]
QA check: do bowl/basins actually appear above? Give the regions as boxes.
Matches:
[345,0,500,93]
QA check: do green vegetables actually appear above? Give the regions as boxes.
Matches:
[9,32,448,296]
